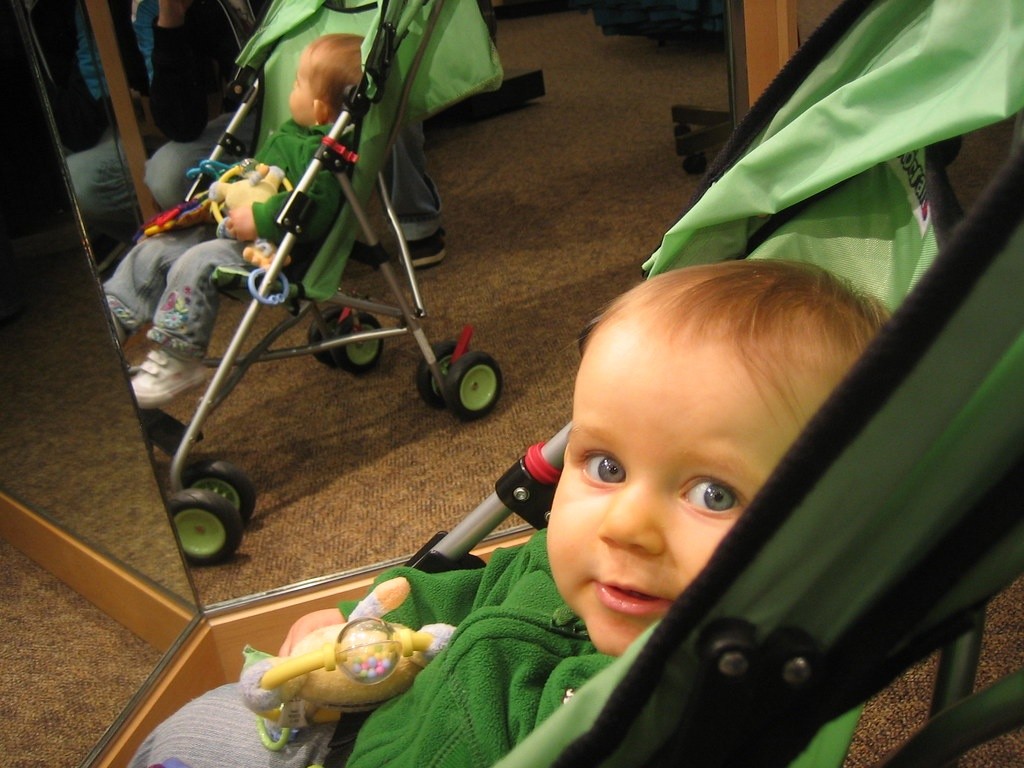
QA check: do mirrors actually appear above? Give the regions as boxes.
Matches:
[0,0,734,632]
[0,0,225,767]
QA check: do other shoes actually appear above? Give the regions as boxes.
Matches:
[406,226,447,270]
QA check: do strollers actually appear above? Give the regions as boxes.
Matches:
[400,0,1023,768]
[122,1,503,568]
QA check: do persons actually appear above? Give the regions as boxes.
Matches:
[65,0,447,410]
[128,259,895,768]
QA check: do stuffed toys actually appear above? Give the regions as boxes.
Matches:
[241,577,459,753]
[208,158,294,305]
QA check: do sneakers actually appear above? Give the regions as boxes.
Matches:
[130,346,208,409]
